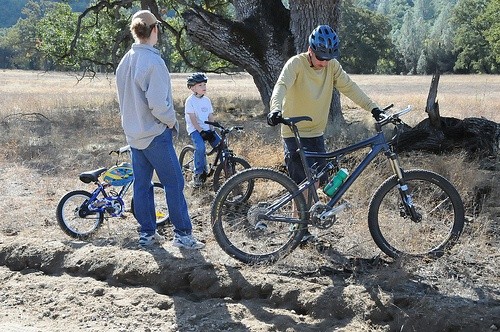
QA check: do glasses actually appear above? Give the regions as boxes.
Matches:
[315,54,333,61]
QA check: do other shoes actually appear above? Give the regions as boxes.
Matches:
[287,228,315,244]
[226,170,237,177]
[193,175,205,188]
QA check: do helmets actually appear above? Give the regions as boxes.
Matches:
[187,73,207,86]
[103,164,134,186]
[309,25,339,60]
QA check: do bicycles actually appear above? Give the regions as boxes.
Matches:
[210,103,465,266]
[179,120,255,208]
[56,144,170,241]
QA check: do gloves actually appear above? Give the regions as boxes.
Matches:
[371,107,386,121]
[266,110,282,126]
[201,130,216,143]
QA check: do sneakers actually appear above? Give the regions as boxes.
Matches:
[172,233,205,249]
[139,229,165,245]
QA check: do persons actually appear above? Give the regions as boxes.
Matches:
[185,73,230,187]
[266,25,385,243]
[115,10,206,250]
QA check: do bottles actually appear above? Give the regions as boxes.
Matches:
[323,168,349,198]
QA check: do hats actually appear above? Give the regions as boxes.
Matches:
[132,10,163,26]
